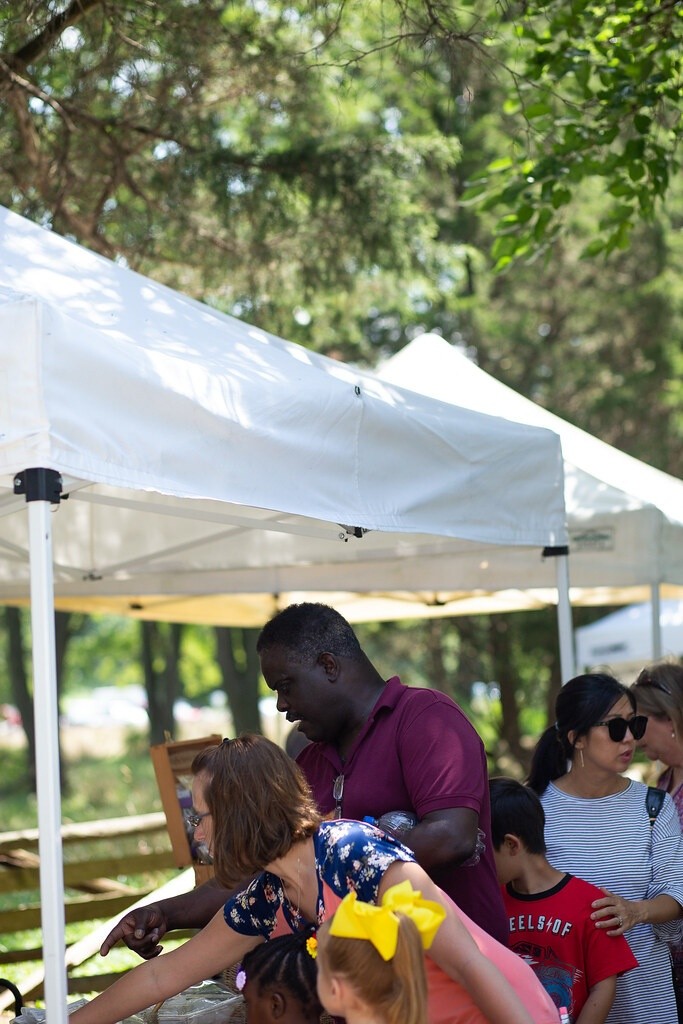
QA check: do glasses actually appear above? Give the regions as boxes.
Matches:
[633,668,672,695]
[186,812,212,827]
[332,772,344,820]
[593,715,647,742]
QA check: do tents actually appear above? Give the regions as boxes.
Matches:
[0,332,683,685]
[0,207,574,1022]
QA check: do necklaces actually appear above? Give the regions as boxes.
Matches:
[280,844,300,917]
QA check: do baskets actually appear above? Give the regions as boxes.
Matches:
[9,959,343,1024]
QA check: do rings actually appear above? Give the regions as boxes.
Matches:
[616,915,623,927]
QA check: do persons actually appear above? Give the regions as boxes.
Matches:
[315,879,447,1023]
[489,779,640,1024]
[235,924,346,1024]
[100,603,510,960]
[67,733,563,1024]
[627,664,683,826]
[524,673,683,1024]
